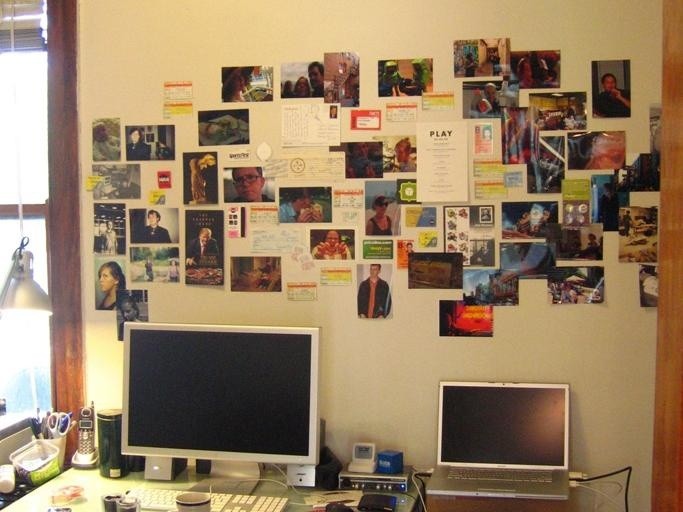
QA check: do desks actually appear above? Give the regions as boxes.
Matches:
[0,465,422,512]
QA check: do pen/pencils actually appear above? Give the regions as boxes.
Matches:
[27,413,71,439]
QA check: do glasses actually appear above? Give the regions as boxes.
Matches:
[232,174,260,184]
[377,202,388,207]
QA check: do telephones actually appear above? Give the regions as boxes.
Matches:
[71,400,99,470]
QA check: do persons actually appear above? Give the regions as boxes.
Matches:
[281,62,357,118]
[312,230,352,260]
[95,122,275,340]
[279,187,324,222]
[358,264,392,319]
[347,142,376,178]
[378,58,429,97]
[406,241,413,256]
[366,194,392,235]
[593,74,631,118]
[389,137,417,172]
[464,54,560,119]
[425,236,437,247]
[222,67,255,102]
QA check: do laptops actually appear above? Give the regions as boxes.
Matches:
[425,381,570,500]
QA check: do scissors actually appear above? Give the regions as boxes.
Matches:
[48,412,71,438]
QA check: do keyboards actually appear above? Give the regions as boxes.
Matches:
[120,489,290,512]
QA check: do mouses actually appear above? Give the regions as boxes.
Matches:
[324,501,355,512]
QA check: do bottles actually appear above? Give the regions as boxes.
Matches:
[98,409,128,478]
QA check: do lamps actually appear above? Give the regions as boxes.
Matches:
[0,2,54,320]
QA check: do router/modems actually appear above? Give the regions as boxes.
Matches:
[357,494,397,512]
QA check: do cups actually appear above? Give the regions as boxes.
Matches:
[175,493,210,512]
[31,435,66,469]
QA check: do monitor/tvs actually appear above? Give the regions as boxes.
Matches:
[120,321,319,492]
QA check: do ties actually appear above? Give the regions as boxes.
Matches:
[202,244,207,256]
